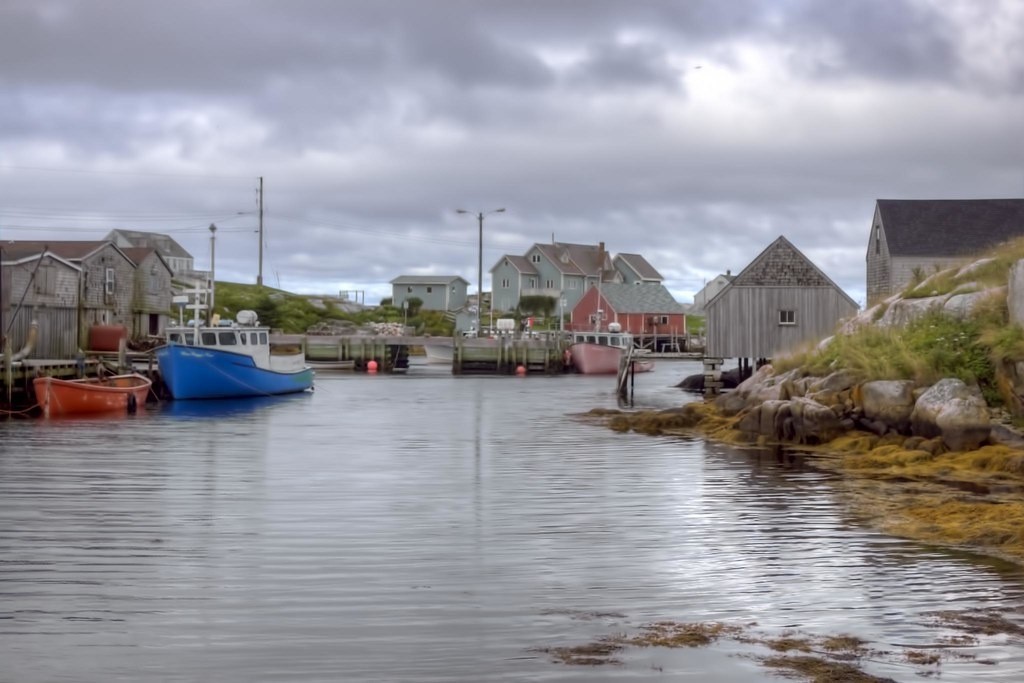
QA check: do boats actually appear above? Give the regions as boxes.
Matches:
[31,371,152,420]
[156,310,317,402]
[569,342,654,374]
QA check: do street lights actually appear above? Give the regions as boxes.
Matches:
[456,206,508,336]
